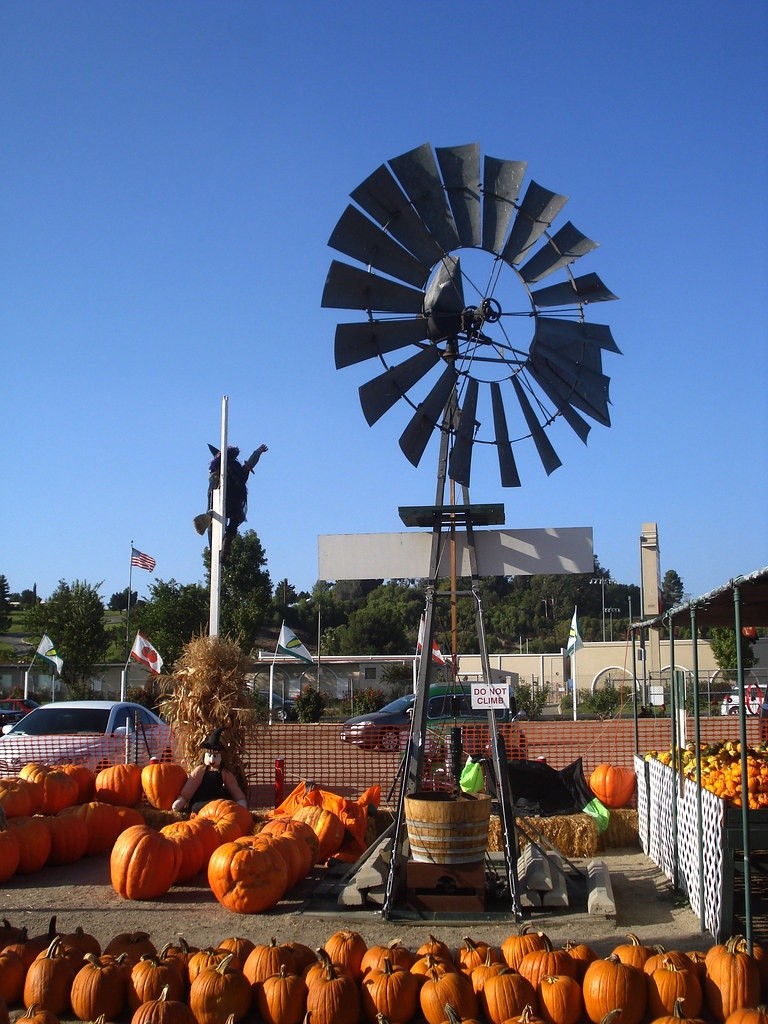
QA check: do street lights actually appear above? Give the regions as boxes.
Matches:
[601,606,621,641]
[589,576,615,642]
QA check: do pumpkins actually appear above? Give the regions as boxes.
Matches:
[0,762,345,914]
[589,764,636,809]
[643,739,768,810]
[0,915,768,1024]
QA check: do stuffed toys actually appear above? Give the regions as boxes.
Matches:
[171,727,246,814]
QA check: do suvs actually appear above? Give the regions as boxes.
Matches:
[720,684,768,717]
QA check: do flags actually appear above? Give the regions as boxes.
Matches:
[131,631,164,676]
[278,625,313,664]
[566,613,584,658]
[36,634,64,675]
[418,616,445,664]
[130,547,156,573]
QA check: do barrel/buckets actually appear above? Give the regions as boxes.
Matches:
[405,790,492,864]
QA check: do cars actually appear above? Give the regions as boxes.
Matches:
[0,687,41,737]
[252,690,300,722]
[0,701,179,780]
[339,692,416,754]
[397,680,528,790]
